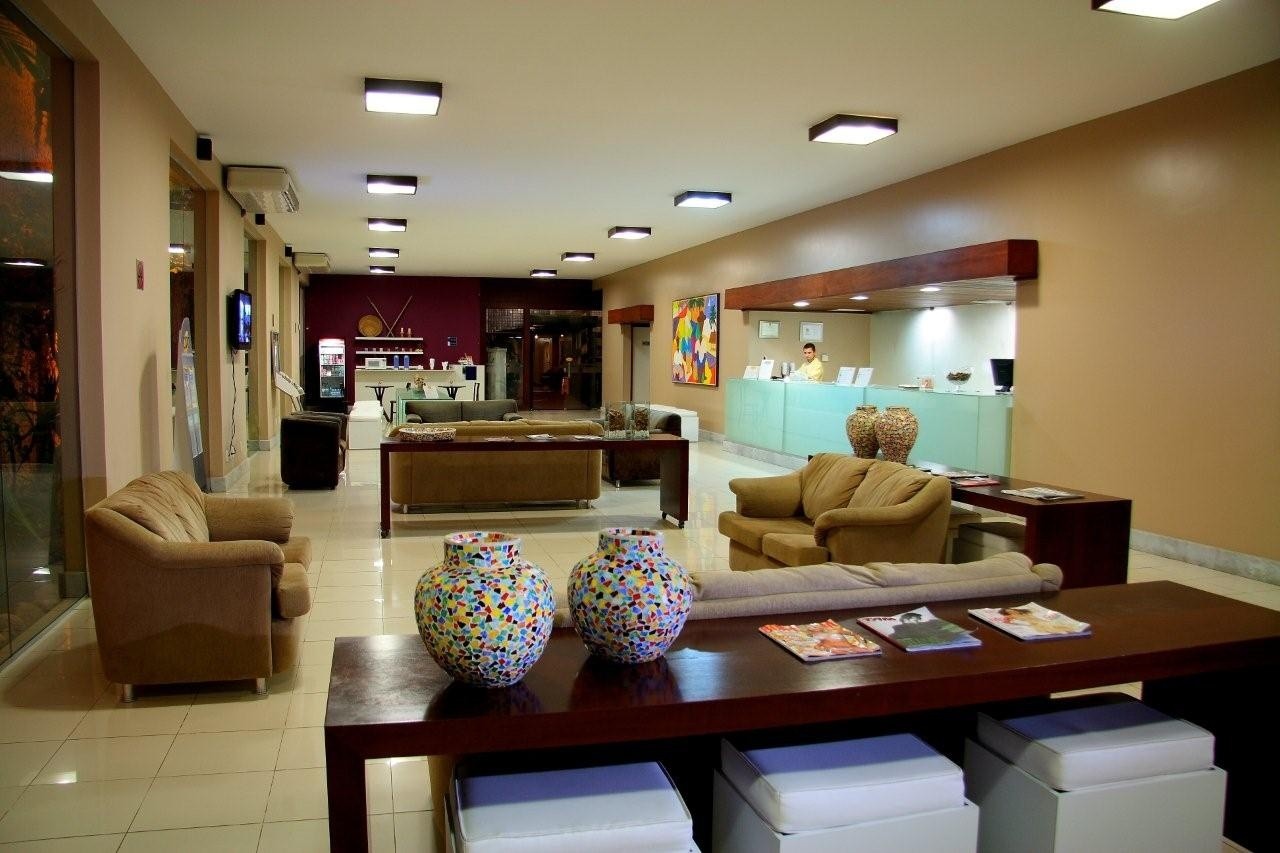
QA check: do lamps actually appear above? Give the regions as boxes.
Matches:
[1087,0,1223,21]
[364,77,443,117]
[366,173,418,197]
[369,266,395,274]
[530,269,558,278]
[807,113,899,148]
[673,190,733,210]
[368,217,408,232]
[0,161,54,186]
[560,251,596,264]
[368,248,400,259]
[607,225,653,241]
[169,243,192,254]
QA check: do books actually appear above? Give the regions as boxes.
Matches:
[967,602,1092,641]
[526,433,557,440]
[945,471,1001,486]
[759,619,884,663]
[573,434,602,441]
[485,436,514,442]
[1002,486,1085,501]
[856,606,984,651]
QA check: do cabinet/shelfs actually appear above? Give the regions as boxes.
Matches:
[354,334,427,373]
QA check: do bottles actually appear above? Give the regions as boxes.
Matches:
[567,527,694,665]
[847,406,880,457]
[394,355,399,371]
[875,406,918,464]
[404,356,409,370]
[416,532,557,690]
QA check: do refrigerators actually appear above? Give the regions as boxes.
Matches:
[315,338,347,412]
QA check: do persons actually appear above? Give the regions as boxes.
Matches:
[999,607,1072,634]
[796,343,823,382]
[887,612,922,638]
[808,624,872,655]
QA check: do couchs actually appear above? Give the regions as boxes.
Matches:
[81,464,312,701]
[717,452,952,581]
[388,400,681,514]
[279,410,349,491]
[468,550,1071,630]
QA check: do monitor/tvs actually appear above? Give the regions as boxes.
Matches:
[227,289,253,350]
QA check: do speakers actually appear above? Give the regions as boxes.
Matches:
[255,213,266,225]
[285,246,292,257]
[197,138,212,161]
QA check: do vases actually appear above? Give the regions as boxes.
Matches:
[410,526,695,690]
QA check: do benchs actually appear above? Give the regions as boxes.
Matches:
[636,402,700,442]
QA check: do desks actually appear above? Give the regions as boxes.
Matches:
[379,433,690,540]
[363,382,390,426]
[318,577,1279,853]
[437,384,467,402]
[807,453,1135,589]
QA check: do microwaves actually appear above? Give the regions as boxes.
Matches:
[365,358,386,369]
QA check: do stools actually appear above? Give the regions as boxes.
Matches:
[347,398,383,450]
[440,691,1231,853]
[942,506,1027,564]
